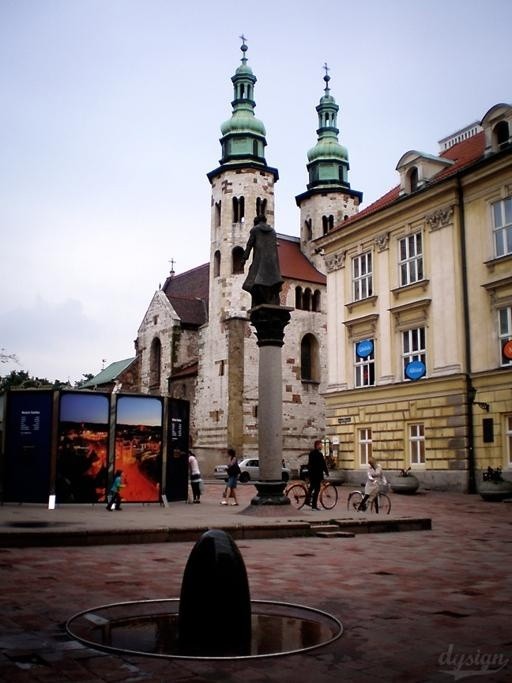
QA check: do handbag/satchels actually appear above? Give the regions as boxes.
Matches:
[226,463,241,478]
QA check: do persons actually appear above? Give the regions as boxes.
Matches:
[220,449,240,506]
[237,214,284,310]
[189,450,202,504]
[304,440,329,510]
[358,456,387,510]
[105,469,126,511]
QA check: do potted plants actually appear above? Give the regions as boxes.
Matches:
[323,455,346,486]
[479,466,512,503]
[389,467,420,495]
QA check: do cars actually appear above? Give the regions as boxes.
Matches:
[298,455,345,484]
[214,458,289,485]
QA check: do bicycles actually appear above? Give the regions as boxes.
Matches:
[286,471,339,510]
[349,477,394,516]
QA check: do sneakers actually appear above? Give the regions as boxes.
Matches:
[311,507,322,512]
[219,498,239,506]
[192,500,201,504]
